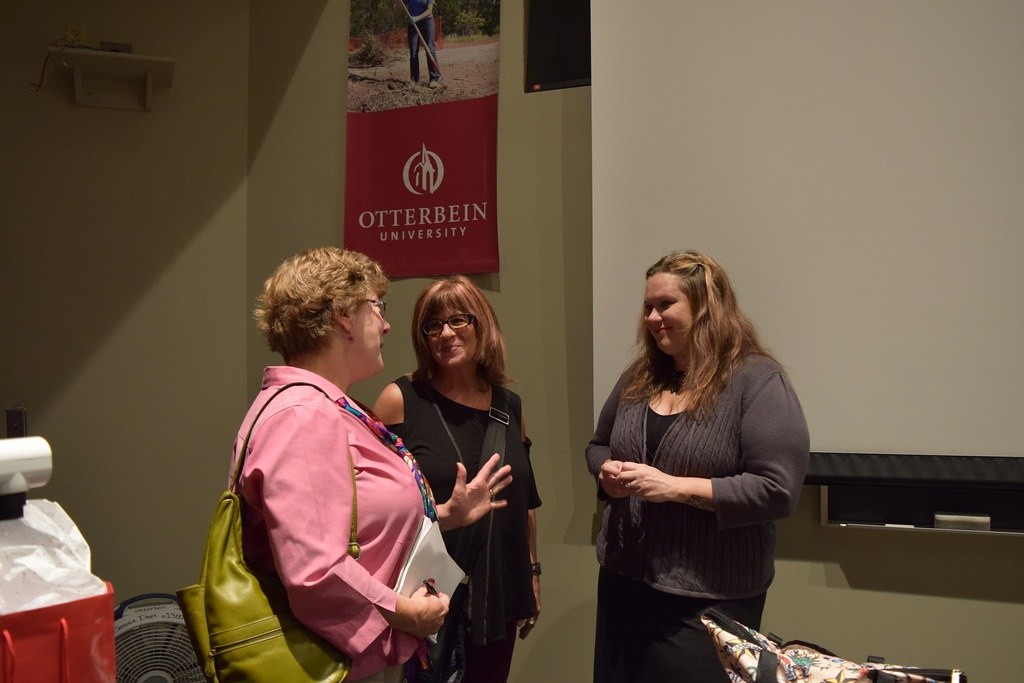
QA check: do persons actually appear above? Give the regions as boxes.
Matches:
[393,0,441,88]
[585,251,810,683]
[374,275,542,683]
[228,250,513,683]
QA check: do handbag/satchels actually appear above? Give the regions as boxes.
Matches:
[0,581,116,683]
[701,607,967,683]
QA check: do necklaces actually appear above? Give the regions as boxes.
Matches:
[675,370,683,380]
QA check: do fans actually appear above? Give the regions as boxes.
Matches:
[114,593,207,683]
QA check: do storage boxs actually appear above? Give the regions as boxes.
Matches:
[0,581,118,683]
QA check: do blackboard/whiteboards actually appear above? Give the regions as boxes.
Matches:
[818,477,1024,538]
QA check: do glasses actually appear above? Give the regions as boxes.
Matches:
[422,313,476,335]
[357,297,386,318]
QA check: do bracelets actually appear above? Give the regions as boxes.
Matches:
[532,563,541,575]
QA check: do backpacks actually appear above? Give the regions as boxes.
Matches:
[175,382,360,683]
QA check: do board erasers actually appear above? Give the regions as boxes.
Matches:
[934,511,991,532]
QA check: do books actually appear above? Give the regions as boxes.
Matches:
[393,515,466,644]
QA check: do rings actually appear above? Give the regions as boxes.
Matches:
[530,621,533,624]
[630,482,631,488]
[490,489,494,497]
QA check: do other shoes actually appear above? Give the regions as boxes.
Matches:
[429,80,438,88]
